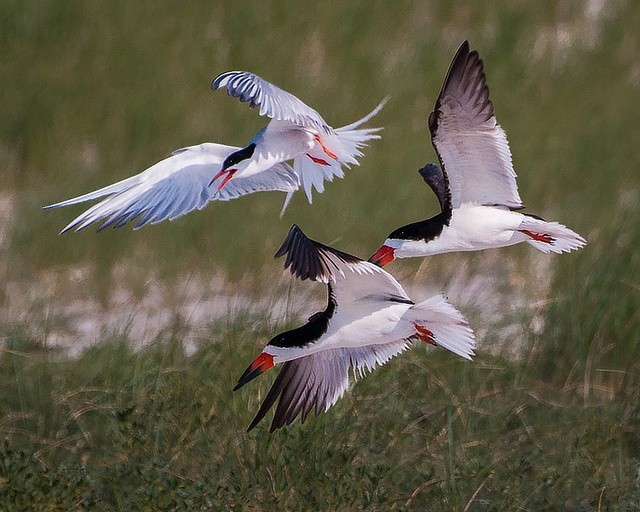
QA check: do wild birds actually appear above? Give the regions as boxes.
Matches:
[367,39,589,269]
[230,223,477,434]
[42,69,392,235]
